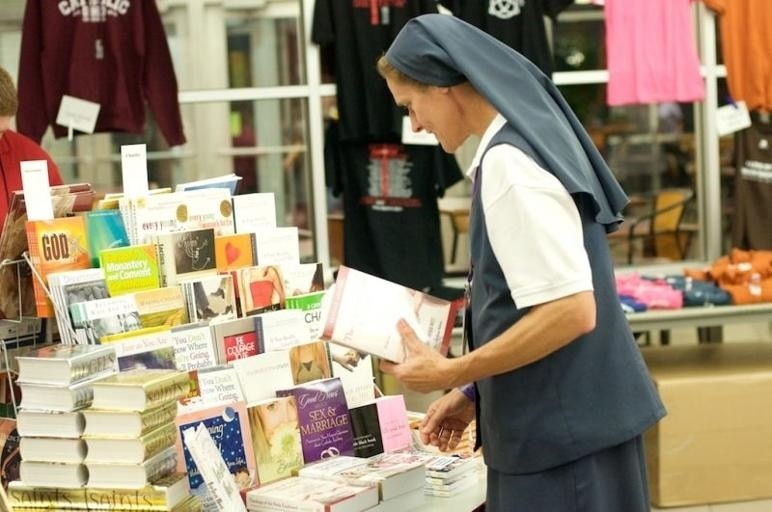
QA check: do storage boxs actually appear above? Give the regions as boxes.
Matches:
[635,344,772,509]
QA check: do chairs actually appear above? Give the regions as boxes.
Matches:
[608,188,694,265]
[441,208,472,265]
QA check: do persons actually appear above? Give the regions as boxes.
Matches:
[0,69,64,228]
[377,14,666,512]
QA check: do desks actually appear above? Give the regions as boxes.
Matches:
[612,265,771,342]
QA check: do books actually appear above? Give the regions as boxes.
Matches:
[2,175,475,511]
